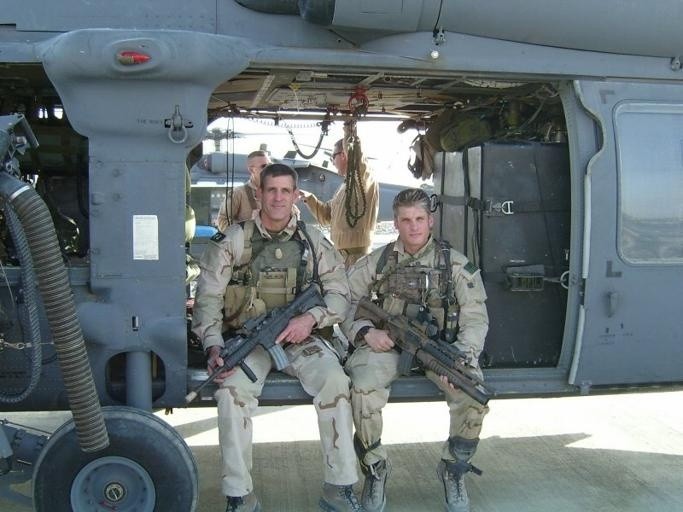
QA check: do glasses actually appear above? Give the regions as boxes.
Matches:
[248,164,266,170]
[330,152,342,161]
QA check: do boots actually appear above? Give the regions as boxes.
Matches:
[318,457,392,512]
[436,459,470,512]
[225,491,261,512]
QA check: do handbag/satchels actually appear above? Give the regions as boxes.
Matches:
[424,108,491,152]
[224,285,267,327]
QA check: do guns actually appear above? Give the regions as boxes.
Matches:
[354,296,497,408]
[184,282,329,403]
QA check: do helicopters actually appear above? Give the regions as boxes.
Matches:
[186,128,436,229]
[0,1,683,512]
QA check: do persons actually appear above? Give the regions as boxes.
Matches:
[189,164,366,512]
[339,188,490,511]
[295,140,380,268]
[217,152,301,237]
